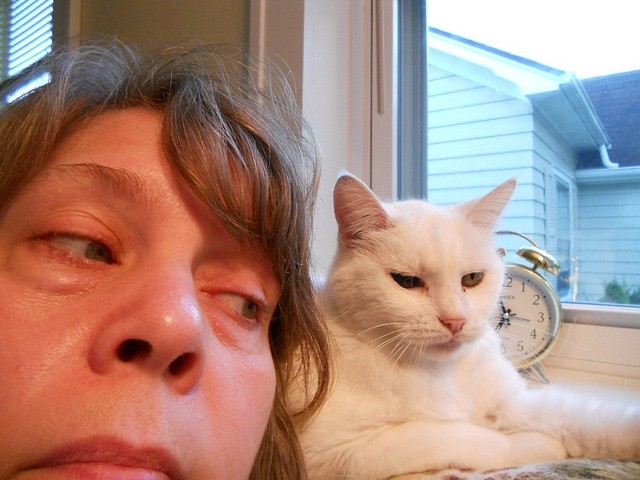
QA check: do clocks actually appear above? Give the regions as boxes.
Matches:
[485,229,561,388]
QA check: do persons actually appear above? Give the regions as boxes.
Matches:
[1,28,343,476]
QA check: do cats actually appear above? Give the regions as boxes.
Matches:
[278,174,640,479]
